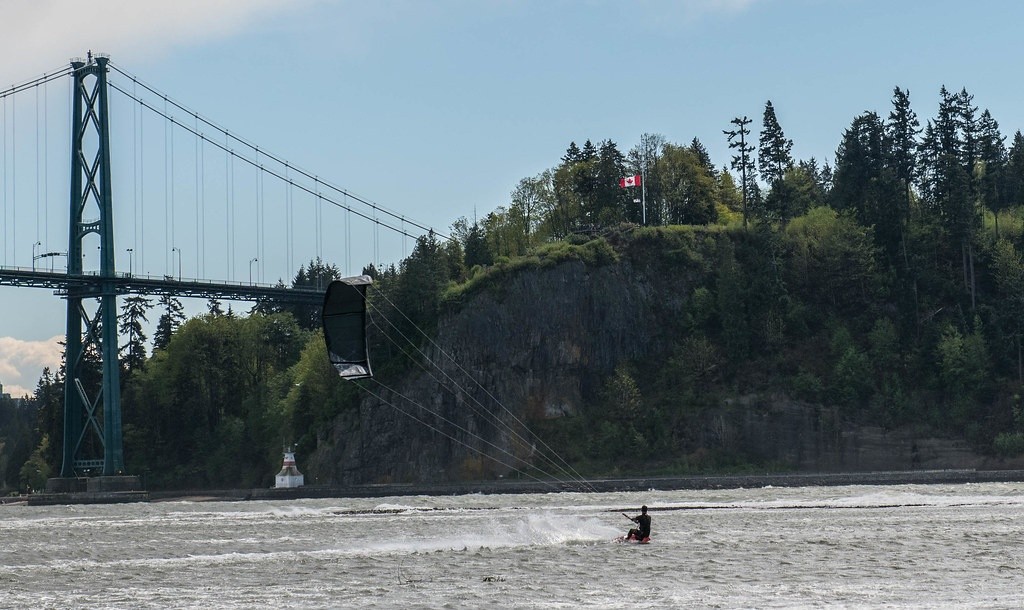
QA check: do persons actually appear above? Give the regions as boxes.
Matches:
[625,506,651,541]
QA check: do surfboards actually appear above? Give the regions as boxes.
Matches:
[619,534,649,544]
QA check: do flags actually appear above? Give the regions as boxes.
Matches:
[621,175,641,187]
